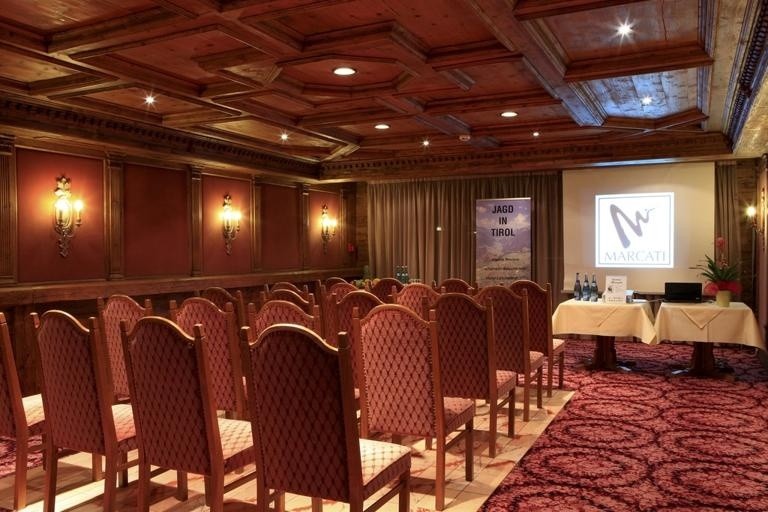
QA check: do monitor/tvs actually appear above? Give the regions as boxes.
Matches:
[664,282,702,303]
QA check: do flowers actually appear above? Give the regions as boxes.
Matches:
[689,237,746,294]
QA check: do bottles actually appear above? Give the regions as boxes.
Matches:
[396,264,409,284]
[574,272,598,303]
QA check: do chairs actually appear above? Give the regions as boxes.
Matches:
[467,285,544,425]
[97,294,154,405]
[499,279,569,398]
[239,323,412,510]
[167,298,251,473]
[348,301,476,512]
[331,291,386,425]
[193,275,478,306]
[0,308,47,511]
[390,283,447,323]
[117,316,252,512]
[245,300,322,341]
[420,294,516,471]
[28,305,140,512]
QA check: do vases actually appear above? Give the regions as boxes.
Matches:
[716,290,730,307]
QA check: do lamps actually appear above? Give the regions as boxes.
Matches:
[53,176,82,258]
[321,204,336,255]
[223,195,240,255]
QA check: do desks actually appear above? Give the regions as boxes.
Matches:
[654,302,768,377]
[552,299,655,373]
